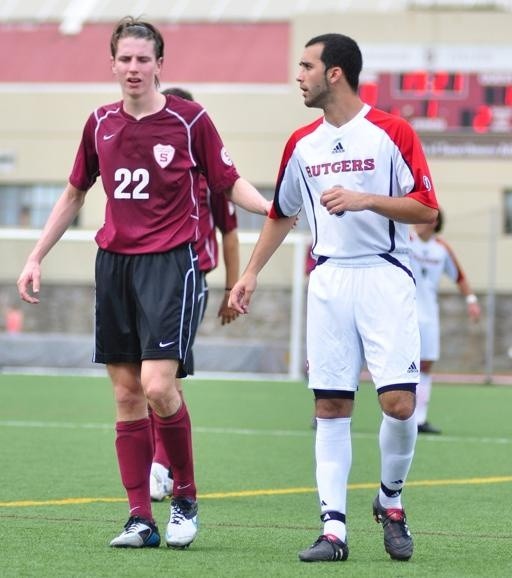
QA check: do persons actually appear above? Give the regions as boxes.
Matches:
[409,210,480,432]
[17,22,299,547]
[148,89,239,501]
[228,33,438,561]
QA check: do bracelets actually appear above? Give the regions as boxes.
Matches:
[225,287,232,291]
[464,295,478,303]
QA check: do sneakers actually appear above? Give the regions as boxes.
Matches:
[150,462,174,501]
[109,516,161,547]
[373,493,413,561]
[418,422,442,433]
[298,534,349,561]
[165,496,198,549]
